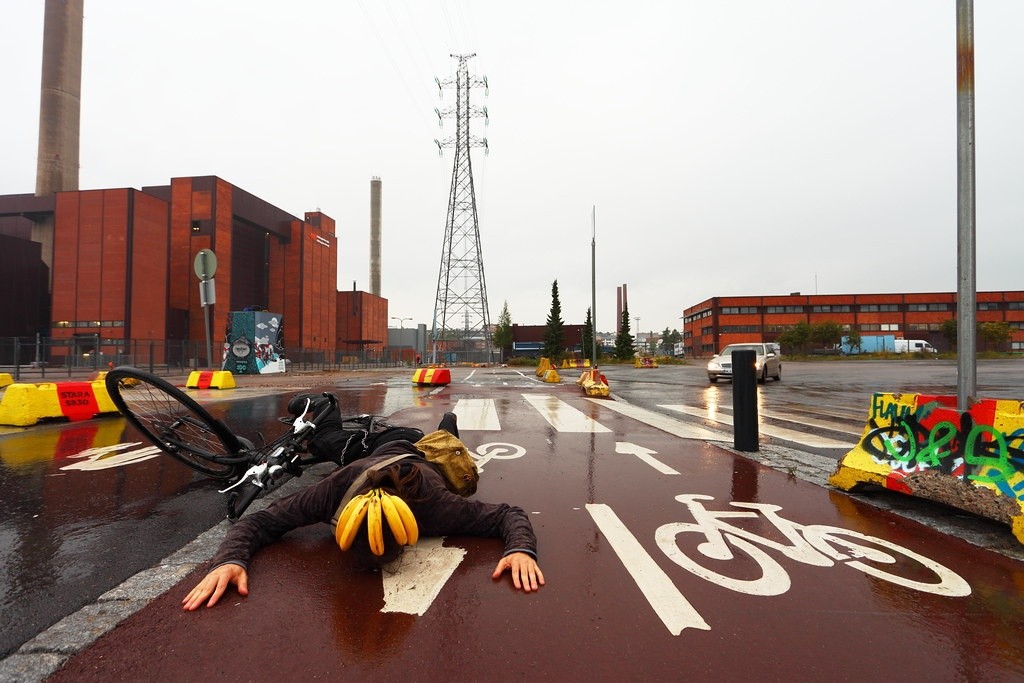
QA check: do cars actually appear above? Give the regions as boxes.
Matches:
[707,342,782,383]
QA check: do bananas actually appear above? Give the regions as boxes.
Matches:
[336,489,418,555]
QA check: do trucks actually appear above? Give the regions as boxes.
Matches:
[894,339,937,354]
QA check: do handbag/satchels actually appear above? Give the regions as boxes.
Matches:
[415,427,481,496]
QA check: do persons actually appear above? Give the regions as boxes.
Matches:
[183,391,545,612]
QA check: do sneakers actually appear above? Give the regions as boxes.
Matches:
[286,391,341,416]
[443,412,457,427]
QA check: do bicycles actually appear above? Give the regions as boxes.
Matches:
[103,365,338,526]
